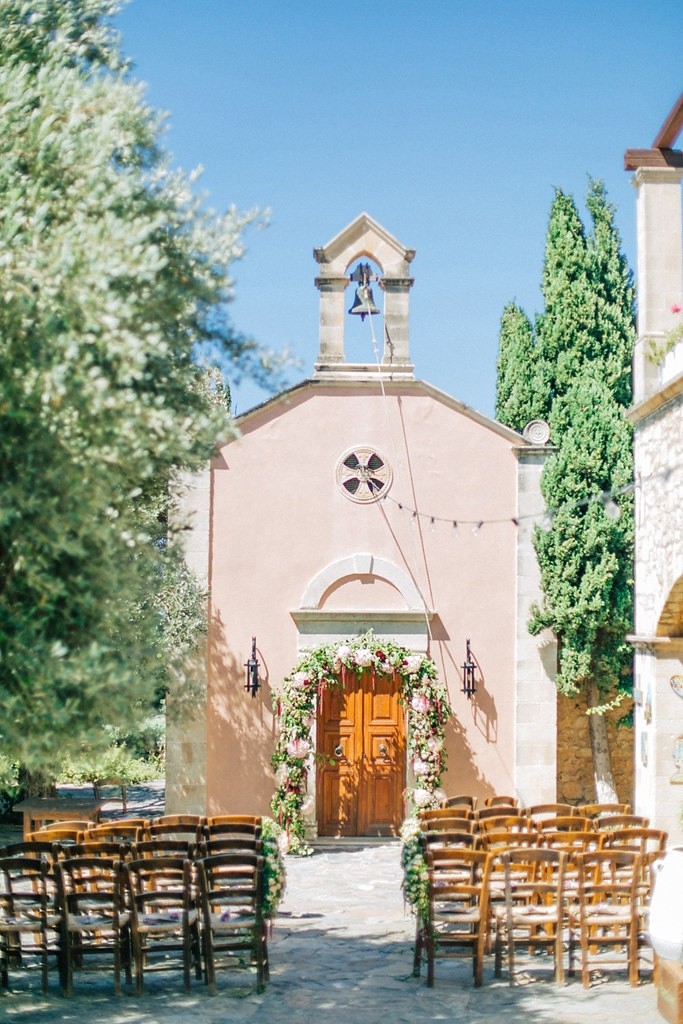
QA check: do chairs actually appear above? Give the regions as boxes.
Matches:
[413,796,668,989]
[0,814,270,997]
[92,777,127,820]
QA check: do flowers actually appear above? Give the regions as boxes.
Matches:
[271,628,452,856]
[252,815,287,991]
[644,303,683,366]
[399,803,438,982]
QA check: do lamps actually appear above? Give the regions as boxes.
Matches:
[244,637,261,698]
[461,639,477,699]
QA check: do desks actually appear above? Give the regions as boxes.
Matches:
[13,797,109,876]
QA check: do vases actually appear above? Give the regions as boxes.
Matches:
[658,339,683,385]
